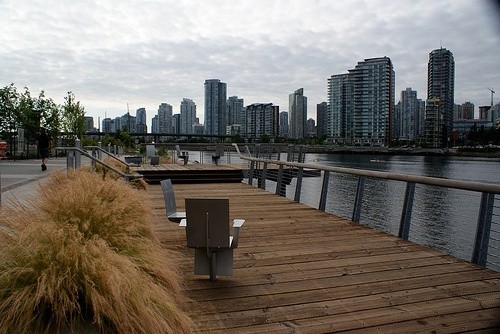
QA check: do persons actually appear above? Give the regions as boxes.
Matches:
[38,127,51,171]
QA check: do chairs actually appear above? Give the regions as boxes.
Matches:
[210,143,225,165]
[175,144,189,165]
[146,144,160,167]
[178,197,245,282]
[161,179,186,223]
